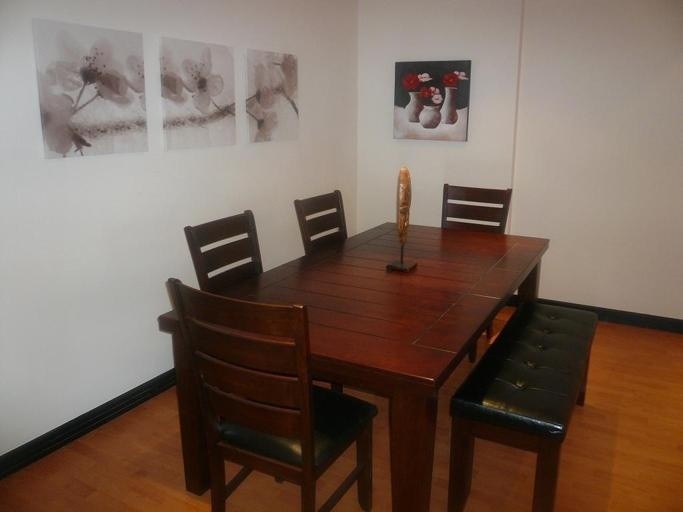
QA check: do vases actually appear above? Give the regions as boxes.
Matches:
[440,87,458,124]
[419,105,442,128]
[404,92,424,122]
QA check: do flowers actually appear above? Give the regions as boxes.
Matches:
[442,70,468,87]
[403,73,433,90]
[420,86,443,104]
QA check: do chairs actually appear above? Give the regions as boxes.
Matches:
[294,190,348,255]
[166,278,379,512]
[185,210,263,294]
[440,183,512,234]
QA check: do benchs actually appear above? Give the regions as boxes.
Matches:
[448,301,598,512]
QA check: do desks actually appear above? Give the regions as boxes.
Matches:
[158,223,550,512]
